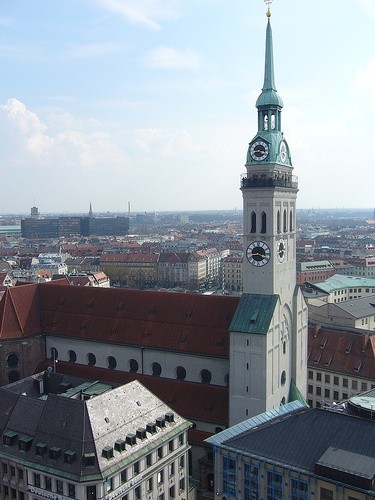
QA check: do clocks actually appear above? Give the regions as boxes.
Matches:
[279,141,288,164]
[249,139,270,162]
[276,237,286,263]
[246,241,271,267]
